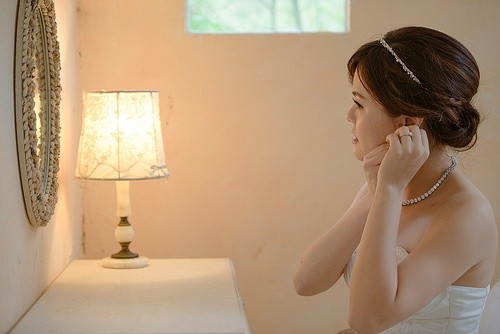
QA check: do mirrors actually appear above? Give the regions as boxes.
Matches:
[14,0,61,228]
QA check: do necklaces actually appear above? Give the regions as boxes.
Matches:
[401,156,456,206]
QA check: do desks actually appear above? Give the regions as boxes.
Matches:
[8,257,251,334]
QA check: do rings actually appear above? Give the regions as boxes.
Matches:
[400,132,412,137]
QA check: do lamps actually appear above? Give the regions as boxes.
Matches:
[74,90,168,269]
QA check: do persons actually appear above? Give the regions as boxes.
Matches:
[293,26,498,334]
